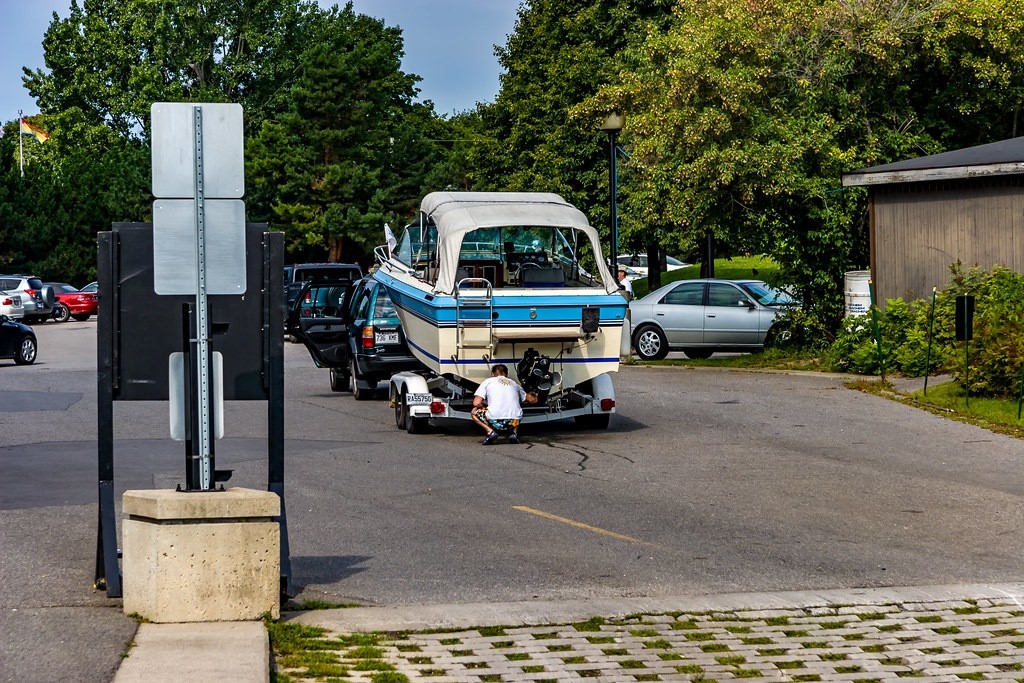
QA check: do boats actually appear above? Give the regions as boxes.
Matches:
[375,192,626,396]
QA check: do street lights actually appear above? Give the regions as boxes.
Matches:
[599,110,625,286]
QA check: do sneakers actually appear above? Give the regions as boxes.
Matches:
[481,431,499,446]
[507,434,520,445]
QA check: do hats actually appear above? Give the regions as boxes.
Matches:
[617,264,629,274]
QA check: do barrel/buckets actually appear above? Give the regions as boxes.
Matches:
[843,271,872,331]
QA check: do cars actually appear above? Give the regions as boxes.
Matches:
[604,252,693,285]
[627,278,807,360]
[0,273,99,365]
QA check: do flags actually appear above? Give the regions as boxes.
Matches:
[21,117,50,145]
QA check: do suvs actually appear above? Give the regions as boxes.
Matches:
[289,278,430,401]
[284,264,362,340]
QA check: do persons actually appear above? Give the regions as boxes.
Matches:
[618,270,634,321]
[471,364,538,445]
